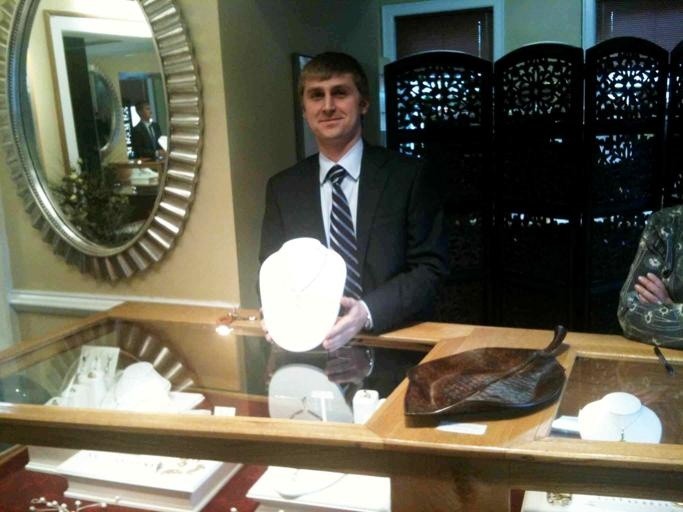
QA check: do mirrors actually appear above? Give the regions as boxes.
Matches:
[1,1,214,291]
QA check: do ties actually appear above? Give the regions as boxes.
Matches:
[323,163,363,325]
[148,124,157,151]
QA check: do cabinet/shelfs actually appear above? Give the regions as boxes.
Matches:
[1,297,683,512]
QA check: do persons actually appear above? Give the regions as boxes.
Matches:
[254,50,443,356]
[594,399,646,444]
[261,337,375,389]
[125,98,163,160]
[615,203,682,351]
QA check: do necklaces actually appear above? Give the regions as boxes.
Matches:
[87,348,104,379]
[76,347,90,374]
[98,349,116,381]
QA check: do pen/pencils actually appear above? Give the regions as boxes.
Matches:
[654,347,673,376]
